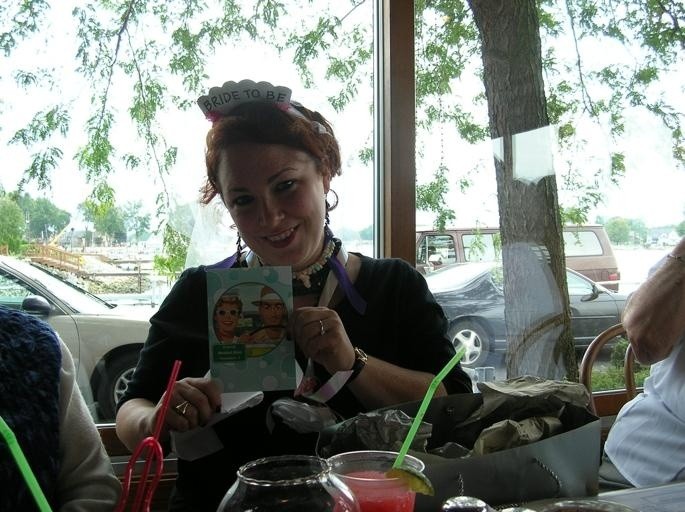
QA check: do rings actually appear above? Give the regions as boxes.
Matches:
[173,399,191,416]
[317,318,329,338]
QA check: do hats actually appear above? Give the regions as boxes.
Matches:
[251,287,285,306]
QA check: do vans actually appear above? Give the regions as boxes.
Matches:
[413,224,622,291]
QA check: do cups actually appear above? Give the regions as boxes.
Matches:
[326,450,425,511]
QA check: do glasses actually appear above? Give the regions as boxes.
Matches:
[216,308,238,316]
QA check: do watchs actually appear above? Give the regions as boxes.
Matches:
[326,345,370,386]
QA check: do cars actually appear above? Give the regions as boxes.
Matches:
[0,257,159,422]
[426,259,629,369]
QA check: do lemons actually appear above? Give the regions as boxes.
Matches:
[386,466,435,496]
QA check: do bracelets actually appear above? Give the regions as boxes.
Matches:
[666,251,685,266]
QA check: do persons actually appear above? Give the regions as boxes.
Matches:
[595,234,684,492]
[0,305,124,512]
[240,283,289,357]
[213,292,247,348]
[113,78,473,512]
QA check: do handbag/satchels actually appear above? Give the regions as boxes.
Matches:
[370,393,602,510]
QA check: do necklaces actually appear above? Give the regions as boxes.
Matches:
[243,235,345,309]
